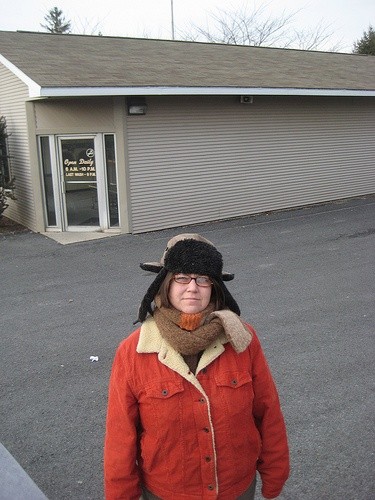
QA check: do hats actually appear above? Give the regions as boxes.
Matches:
[132,232,241,325]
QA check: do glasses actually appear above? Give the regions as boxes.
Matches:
[173,273,215,287]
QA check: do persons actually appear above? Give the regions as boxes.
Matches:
[103,232,290,500]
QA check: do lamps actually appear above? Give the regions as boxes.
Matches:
[127,96,147,115]
